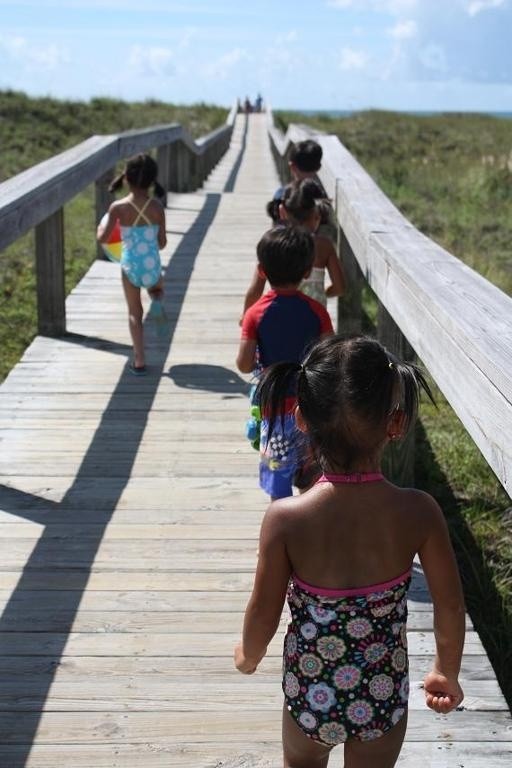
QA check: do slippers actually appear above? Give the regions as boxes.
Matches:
[151,296,170,326]
[128,358,147,377]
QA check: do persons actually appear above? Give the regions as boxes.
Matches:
[273,140,322,224]
[237,226,337,554]
[94,151,167,375]
[235,333,465,766]
[238,178,348,450]
